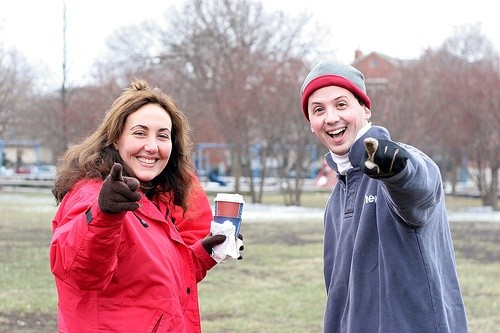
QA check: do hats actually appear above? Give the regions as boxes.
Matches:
[300,61,371,122]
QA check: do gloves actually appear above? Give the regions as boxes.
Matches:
[98,163,141,214]
[359,136,409,180]
[201,232,244,260]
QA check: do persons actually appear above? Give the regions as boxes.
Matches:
[301,58,469,333]
[48,75,244,332]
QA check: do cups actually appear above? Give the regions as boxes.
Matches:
[214,193,244,242]
